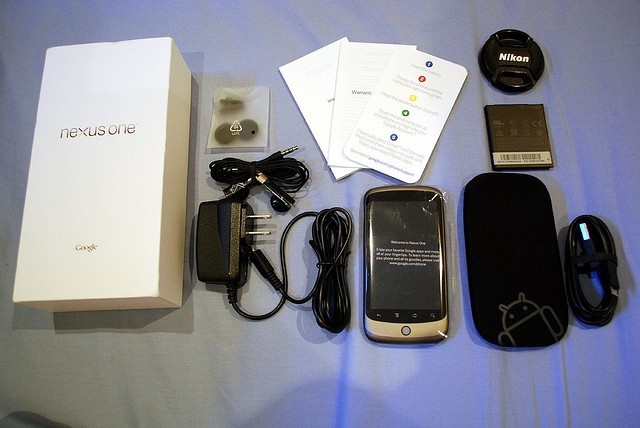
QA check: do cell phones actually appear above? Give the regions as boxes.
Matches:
[362,185,450,343]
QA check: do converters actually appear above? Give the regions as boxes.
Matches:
[193,200,271,289]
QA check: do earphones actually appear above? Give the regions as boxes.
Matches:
[252,173,295,213]
